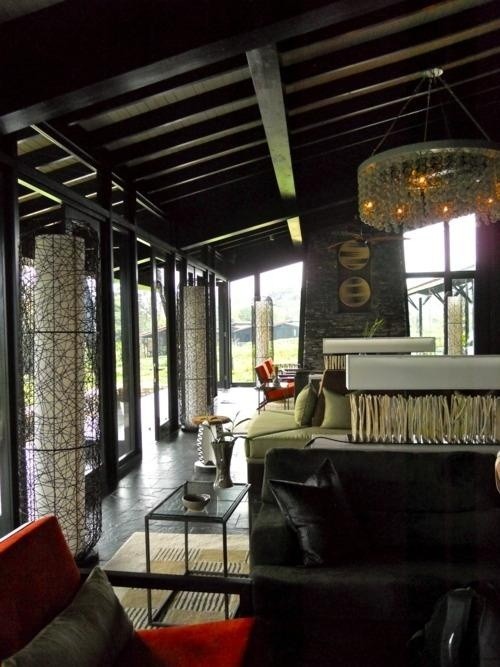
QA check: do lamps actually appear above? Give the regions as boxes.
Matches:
[356,66,500,235]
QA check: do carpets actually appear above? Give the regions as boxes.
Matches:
[101,530,251,630]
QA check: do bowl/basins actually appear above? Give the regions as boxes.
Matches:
[182,494,211,512]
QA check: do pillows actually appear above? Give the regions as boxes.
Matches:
[265,458,364,567]
[1,565,134,667]
[294,383,318,427]
[320,386,352,429]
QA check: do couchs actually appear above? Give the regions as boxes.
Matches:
[248,447,500,667]
[245,369,359,494]
[242,378,324,495]
[0,513,267,667]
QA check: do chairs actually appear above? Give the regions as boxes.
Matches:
[255,364,295,410]
[262,357,296,383]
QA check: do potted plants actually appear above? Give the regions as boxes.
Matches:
[359,315,384,356]
[202,410,252,488]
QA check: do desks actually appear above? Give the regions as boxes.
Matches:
[255,381,289,415]
[144,480,252,626]
[310,433,500,456]
[294,368,323,408]
